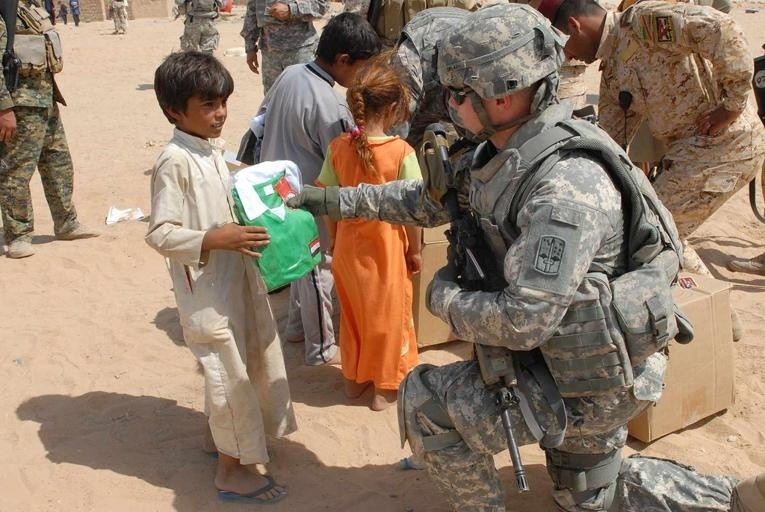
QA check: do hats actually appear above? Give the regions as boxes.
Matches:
[536,0,564,24]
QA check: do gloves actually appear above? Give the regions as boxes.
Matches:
[286,185,339,222]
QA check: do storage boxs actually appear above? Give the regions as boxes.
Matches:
[626,271,734,443]
[403,220,460,348]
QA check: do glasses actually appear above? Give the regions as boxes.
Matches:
[446,86,472,107]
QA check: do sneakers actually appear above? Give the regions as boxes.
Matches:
[8,241,34,257]
[56,224,103,240]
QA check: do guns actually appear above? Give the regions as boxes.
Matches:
[0,1,21,93]
[416,121,529,495]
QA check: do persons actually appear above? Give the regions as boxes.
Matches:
[230,10,382,365]
[173,0,222,55]
[238,0,332,99]
[69,0,81,26]
[285,3,765,511]
[344,1,514,148]
[0,0,101,257]
[142,50,298,504]
[58,1,68,25]
[549,2,763,342]
[109,0,128,35]
[44,0,55,24]
[311,59,427,409]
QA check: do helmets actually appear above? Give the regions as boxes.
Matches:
[437,3,566,100]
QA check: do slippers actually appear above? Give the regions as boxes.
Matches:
[218,475,289,504]
